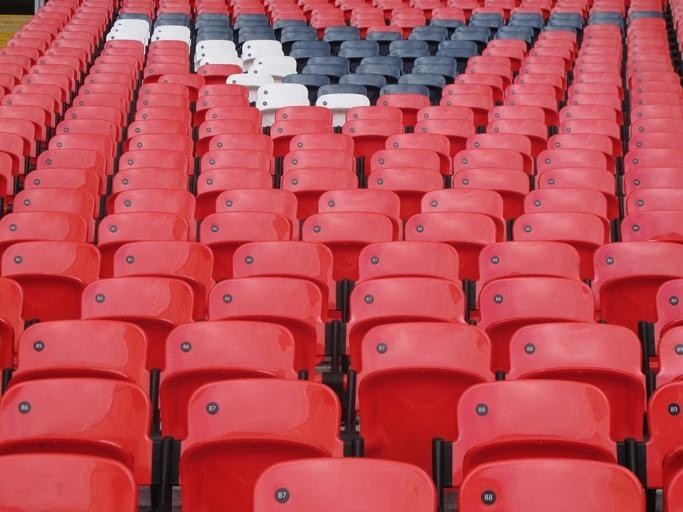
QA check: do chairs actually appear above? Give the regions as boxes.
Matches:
[2,1,683,512]
